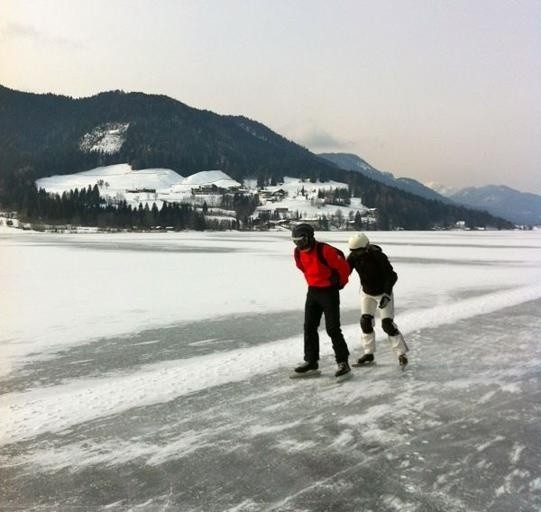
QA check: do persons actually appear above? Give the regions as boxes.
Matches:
[290,222,352,377]
[345,232,409,368]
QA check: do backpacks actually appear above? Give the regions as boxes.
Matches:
[316,240,346,290]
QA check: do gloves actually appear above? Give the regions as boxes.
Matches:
[377,294,392,309]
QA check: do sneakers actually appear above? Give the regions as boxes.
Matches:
[334,359,352,377]
[398,354,408,366]
[294,361,319,373]
[357,353,375,364]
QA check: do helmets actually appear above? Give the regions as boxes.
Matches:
[348,232,369,250]
[291,223,316,252]
